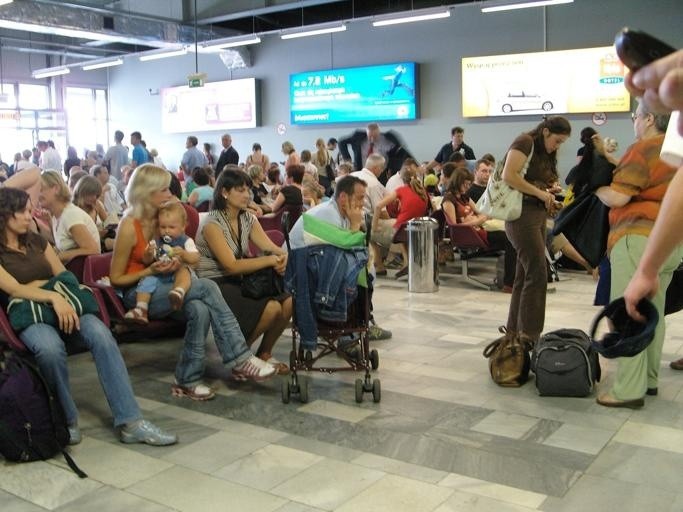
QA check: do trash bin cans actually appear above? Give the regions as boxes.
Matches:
[408,216,440,293]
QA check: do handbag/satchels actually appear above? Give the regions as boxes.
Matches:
[532,327,600,398]
[6,269,102,334]
[240,250,285,300]
[484,325,534,389]
[474,136,534,224]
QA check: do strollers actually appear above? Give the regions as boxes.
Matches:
[274,207,384,407]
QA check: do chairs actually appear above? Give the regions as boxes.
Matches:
[439,217,495,294]
[1,277,111,359]
[254,205,288,228]
[77,255,184,344]
[247,228,283,257]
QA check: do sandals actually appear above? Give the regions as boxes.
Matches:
[167,287,183,313]
[123,305,150,326]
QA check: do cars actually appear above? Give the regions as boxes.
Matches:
[498,88,556,115]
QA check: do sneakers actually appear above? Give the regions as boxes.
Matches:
[366,324,393,341]
[504,286,512,292]
[335,340,361,358]
[170,379,215,401]
[231,353,280,384]
[119,418,177,447]
[68,423,82,446]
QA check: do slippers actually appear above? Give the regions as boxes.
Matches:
[265,356,289,375]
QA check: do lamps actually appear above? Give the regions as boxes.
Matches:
[476,0,579,12]
[136,42,186,62]
[370,0,454,29]
[278,1,346,42]
[81,57,126,73]
[198,15,260,53]
[27,63,72,79]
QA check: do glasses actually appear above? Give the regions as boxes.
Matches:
[631,111,639,122]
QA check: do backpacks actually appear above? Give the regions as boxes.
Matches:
[0,347,77,465]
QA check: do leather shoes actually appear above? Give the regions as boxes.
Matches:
[395,265,408,277]
[670,358,683,371]
[375,270,387,276]
[646,387,658,396]
[596,392,645,410]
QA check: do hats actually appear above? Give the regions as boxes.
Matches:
[588,295,660,359]
[424,173,438,186]
